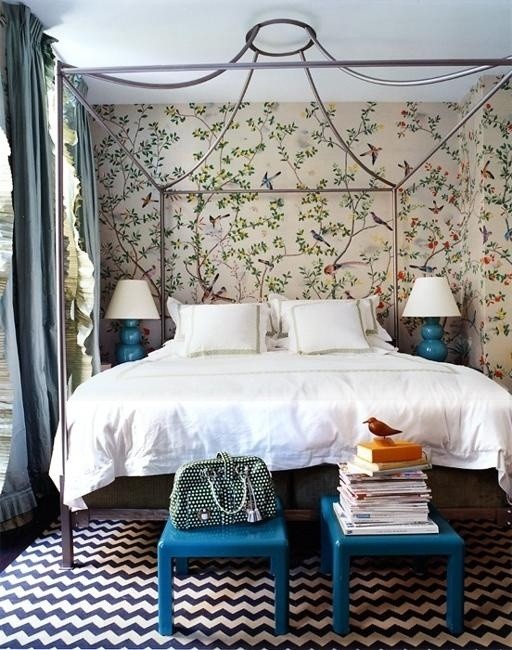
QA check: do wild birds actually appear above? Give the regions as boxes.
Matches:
[311,230,330,247]
[202,274,227,304]
[479,226,492,244]
[398,161,414,177]
[140,265,156,280]
[142,193,158,208]
[324,261,369,275]
[363,417,403,439]
[209,215,221,228]
[259,259,274,272]
[260,172,282,189]
[360,143,382,165]
[482,160,495,180]
[429,200,444,214]
[371,212,393,230]
[409,265,438,272]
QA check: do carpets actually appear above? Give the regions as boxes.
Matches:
[0,512,512,650]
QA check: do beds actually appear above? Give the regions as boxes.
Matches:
[47,336,512,571]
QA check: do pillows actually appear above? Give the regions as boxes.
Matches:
[163,292,399,361]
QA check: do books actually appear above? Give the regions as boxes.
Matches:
[333,441,439,535]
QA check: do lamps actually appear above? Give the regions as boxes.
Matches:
[104,280,161,364]
[402,277,461,362]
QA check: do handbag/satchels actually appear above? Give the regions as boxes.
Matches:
[168,451,278,530]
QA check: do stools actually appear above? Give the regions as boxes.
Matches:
[158,515,289,636]
[319,496,466,635]
